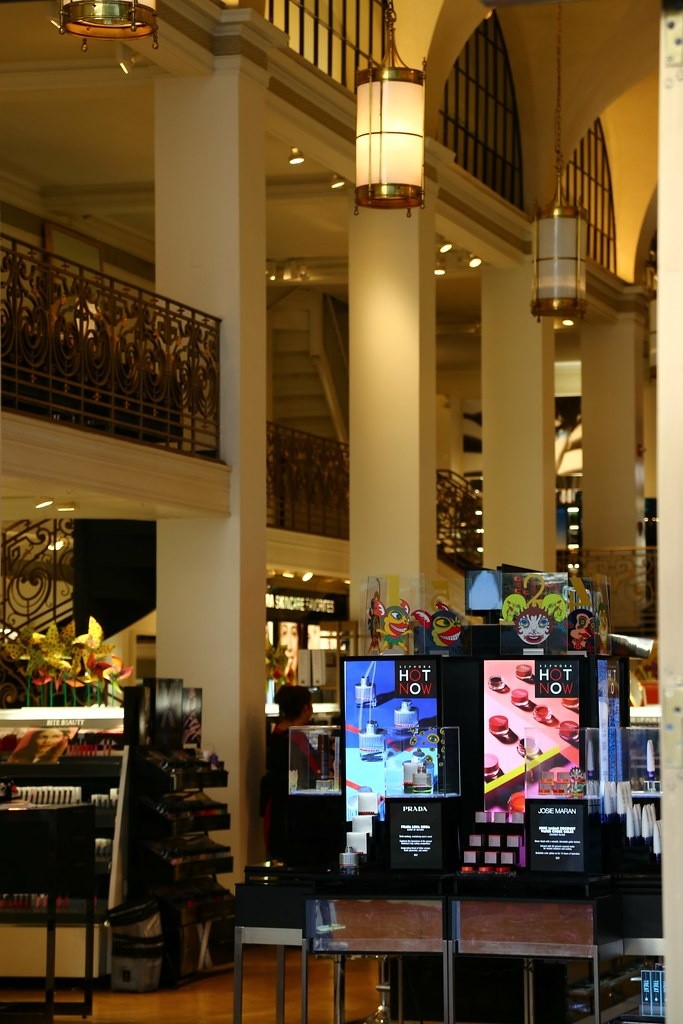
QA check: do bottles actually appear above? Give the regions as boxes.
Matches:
[414,765,432,791]
[316,774,333,791]
[402,750,434,787]
[339,845,359,869]
[393,701,419,736]
[359,721,386,759]
[355,676,377,708]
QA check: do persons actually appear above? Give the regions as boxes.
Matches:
[12,728,70,763]
[259,684,344,867]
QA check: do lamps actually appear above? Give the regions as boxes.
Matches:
[528,0,589,321]
[288,146,306,165]
[331,172,345,189]
[264,258,307,280]
[433,232,482,275]
[58,0,159,52]
[353,0,427,218]
[644,284,658,382]
